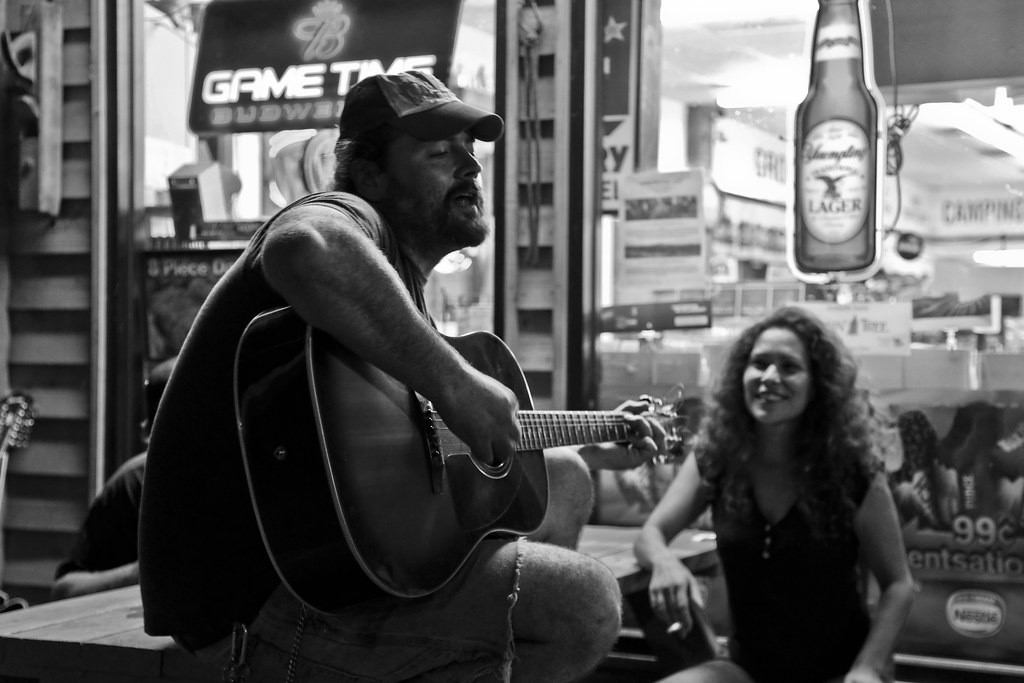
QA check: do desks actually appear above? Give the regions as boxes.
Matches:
[0,524,719,683]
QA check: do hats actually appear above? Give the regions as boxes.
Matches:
[341,71,503,143]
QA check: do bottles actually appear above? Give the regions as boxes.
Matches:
[794,0,879,274]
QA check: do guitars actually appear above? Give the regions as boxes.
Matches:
[230,303,682,618]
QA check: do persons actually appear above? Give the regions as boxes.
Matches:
[51,356,180,601]
[633,306,915,683]
[860,388,1024,539]
[137,70,668,683]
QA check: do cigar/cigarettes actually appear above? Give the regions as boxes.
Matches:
[668,621,683,634]
